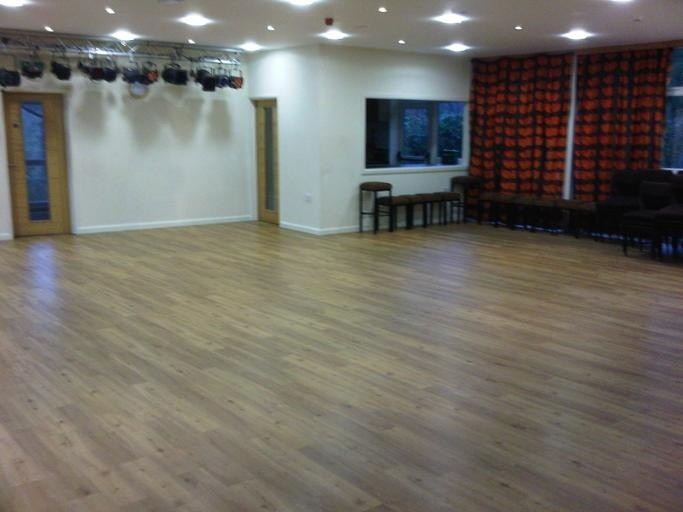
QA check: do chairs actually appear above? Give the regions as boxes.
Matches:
[597,168,683,258]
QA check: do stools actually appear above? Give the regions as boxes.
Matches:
[359,175,596,240]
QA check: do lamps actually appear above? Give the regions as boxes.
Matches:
[0,29,243,92]
